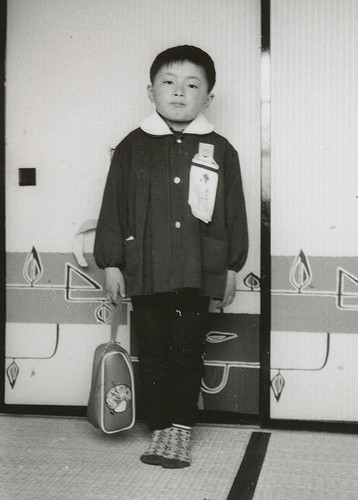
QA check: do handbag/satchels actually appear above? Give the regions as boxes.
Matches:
[86,341,135,435]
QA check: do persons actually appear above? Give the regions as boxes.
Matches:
[93,45,249,469]
